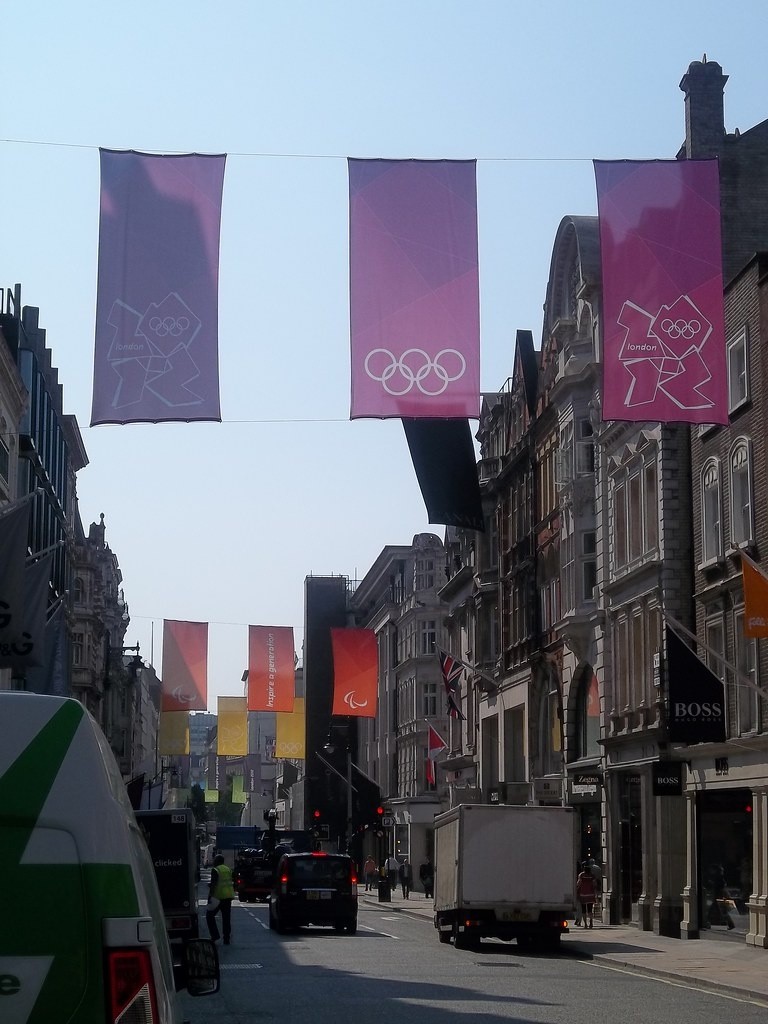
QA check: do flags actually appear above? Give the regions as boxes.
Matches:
[314,756,382,835]
[231,777,247,803]
[427,725,445,785]
[592,159,727,425]
[204,780,219,803]
[740,556,768,639]
[438,645,468,720]
[91,148,226,422]
[154,620,377,789]
[663,625,729,747]
[345,156,484,421]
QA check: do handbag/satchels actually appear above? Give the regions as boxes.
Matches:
[410,881,414,889]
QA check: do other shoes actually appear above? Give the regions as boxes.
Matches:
[365,888,368,891]
[577,924,581,926]
[403,895,405,899]
[211,936,220,942]
[574,922,578,925]
[223,939,230,945]
[369,888,372,891]
[584,924,587,928]
[407,895,409,899]
[589,924,593,928]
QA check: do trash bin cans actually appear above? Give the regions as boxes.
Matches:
[378,881,391,902]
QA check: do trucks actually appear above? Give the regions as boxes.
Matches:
[419,803,579,953]
[211,822,320,906]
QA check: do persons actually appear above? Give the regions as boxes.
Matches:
[364,854,435,900]
[575,859,595,929]
[702,864,735,931]
[590,858,602,902]
[204,854,234,944]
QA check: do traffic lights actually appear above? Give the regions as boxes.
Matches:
[374,801,386,822]
[311,805,321,824]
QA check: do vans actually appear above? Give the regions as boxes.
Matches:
[263,852,359,937]
[0,691,220,1024]
[132,807,205,945]
